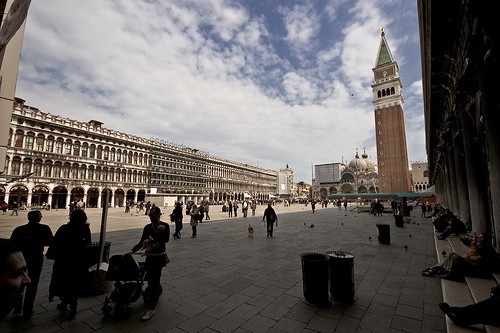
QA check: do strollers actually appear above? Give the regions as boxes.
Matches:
[101,251,148,321]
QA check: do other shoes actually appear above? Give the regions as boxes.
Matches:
[438,301,468,328]
[267,230,272,237]
[142,308,156,320]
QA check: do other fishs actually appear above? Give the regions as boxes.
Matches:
[248,223,253,238]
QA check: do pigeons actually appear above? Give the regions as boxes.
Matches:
[304,221,306,226]
[404,244,408,250]
[409,233,411,237]
[442,249,446,255]
[412,221,415,223]
[369,236,371,238]
[342,222,343,225]
[309,223,314,227]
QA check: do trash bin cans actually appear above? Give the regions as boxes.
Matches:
[299,251,331,306]
[376,222,390,244]
[327,249,359,303]
[406,206,411,217]
[394,214,404,227]
[85,241,112,266]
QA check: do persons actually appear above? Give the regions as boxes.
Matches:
[222,202,256,217]
[421,203,442,217]
[391,200,402,214]
[263,204,277,236]
[432,233,494,279]
[433,208,466,240]
[69,199,85,214]
[45,209,92,318]
[125,199,151,215]
[0,238,31,333]
[129,207,170,319]
[2,200,18,216]
[273,198,347,213]
[173,202,210,238]
[438,284,500,327]
[371,200,384,216]
[10,210,54,316]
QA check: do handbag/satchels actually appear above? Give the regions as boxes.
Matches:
[169,214,175,222]
